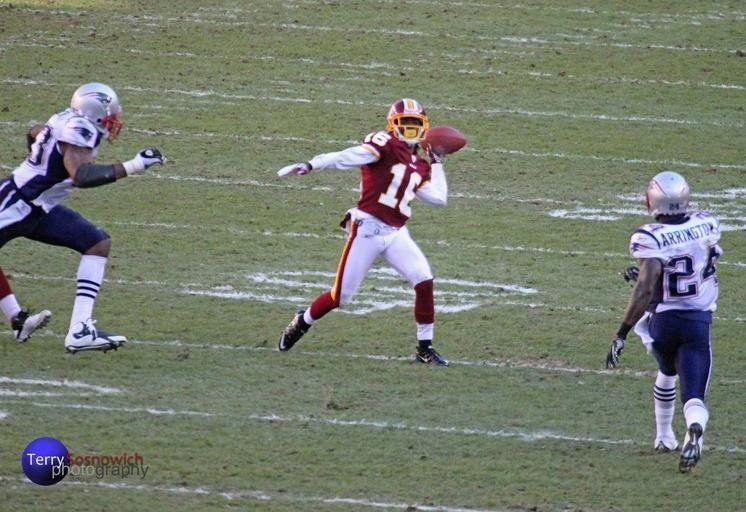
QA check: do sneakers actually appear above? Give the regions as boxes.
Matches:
[278,309,311,351]
[653,438,678,451]
[678,421,703,473]
[64,317,126,354]
[11,309,52,343]
[415,345,449,366]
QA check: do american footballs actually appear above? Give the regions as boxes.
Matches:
[420,126,467,154]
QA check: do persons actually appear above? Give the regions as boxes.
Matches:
[1,264,55,345]
[1,81,176,355]
[602,171,726,474]
[274,96,452,368]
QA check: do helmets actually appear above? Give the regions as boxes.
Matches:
[645,170,690,217]
[386,98,428,143]
[71,82,123,144]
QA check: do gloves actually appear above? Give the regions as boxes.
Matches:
[277,162,312,178]
[426,143,443,164]
[606,321,632,369]
[120,146,166,176]
[624,266,640,282]
